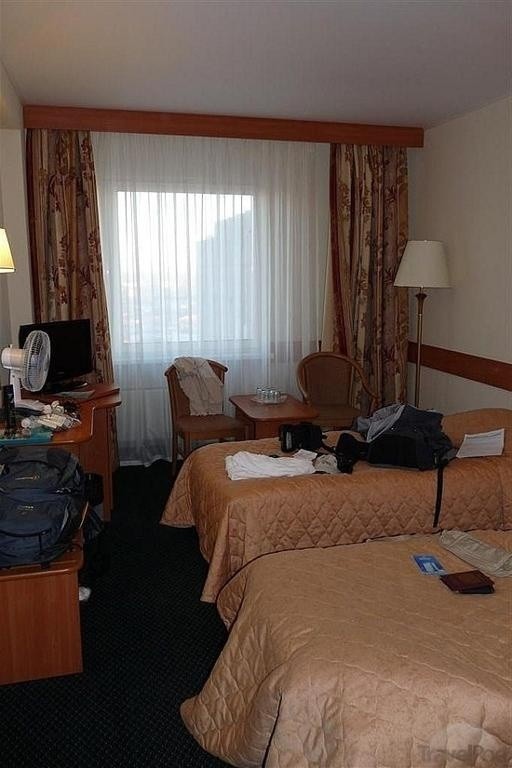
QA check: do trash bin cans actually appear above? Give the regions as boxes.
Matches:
[139,470,169,519]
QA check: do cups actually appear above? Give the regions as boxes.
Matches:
[274,390,280,401]
[261,388,266,399]
[269,388,274,400]
[256,384,260,399]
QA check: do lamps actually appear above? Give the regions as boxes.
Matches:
[393,240,452,408]
[2,226,15,275]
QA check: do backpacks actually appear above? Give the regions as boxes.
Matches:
[0,444,84,572]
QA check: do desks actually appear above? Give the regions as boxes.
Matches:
[0,531,87,685]
[229,392,319,439]
[1,383,123,522]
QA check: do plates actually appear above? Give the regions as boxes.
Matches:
[251,396,287,404]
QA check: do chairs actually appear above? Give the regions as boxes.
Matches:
[164,358,248,477]
[297,351,377,431]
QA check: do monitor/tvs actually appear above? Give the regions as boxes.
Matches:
[18,318,94,391]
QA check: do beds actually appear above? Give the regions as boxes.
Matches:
[160,408,512,605]
[180,529,512,768]
